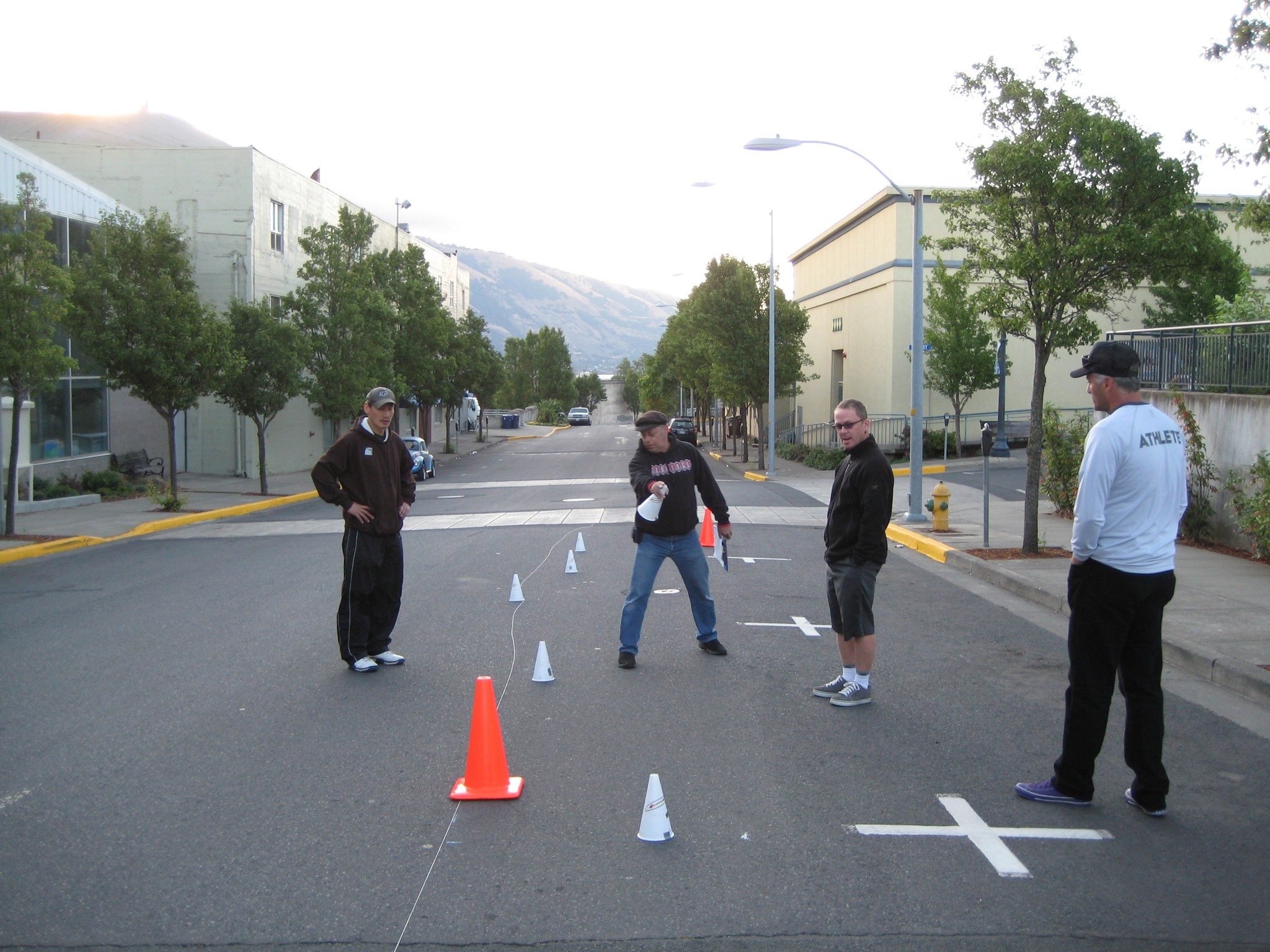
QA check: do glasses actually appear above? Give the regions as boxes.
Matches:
[1082,354,1108,370]
[832,419,863,430]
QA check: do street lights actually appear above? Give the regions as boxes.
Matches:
[743,136,929,523]
[394,198,412,330]
[655,303,696,428]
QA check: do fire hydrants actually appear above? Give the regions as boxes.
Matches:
[924,480,951,531]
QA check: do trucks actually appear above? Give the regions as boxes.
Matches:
[455,396,483,432]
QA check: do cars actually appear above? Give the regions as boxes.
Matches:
[399,436,436,481]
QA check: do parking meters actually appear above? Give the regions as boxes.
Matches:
[943,413,950,461]
[980,423,995,548]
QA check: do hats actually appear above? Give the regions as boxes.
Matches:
[365,386,396,410]
[1070,341,1141,378]
[634,410,668,432]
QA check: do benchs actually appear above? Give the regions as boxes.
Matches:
[979,420,1031,439]
[112,449,164,480]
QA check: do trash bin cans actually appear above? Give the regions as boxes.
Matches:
[512,414,519,428]
[502,414,513,429]
[726,416,741,437]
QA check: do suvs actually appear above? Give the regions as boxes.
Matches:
[666,417,699,447]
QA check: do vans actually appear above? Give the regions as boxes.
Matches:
[568,407,592,426]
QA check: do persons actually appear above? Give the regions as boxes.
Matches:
[311,387,416,671]
[812,399,895,705]
[1015,339,1188,816]
[618,410,732,667]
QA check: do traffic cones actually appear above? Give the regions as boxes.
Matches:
[574,532,587,552]
[530,641,555,682]
[509,574,525,602]
[636,483,668,521]
[698,508,716,547]
[636,773,675,842]
[564,550,578,574]
[449,674,525,800]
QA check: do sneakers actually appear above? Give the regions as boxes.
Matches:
[618,651,637,669]
[813,676,848,698]
[830,681,872,707]
[369,650,406,666]
[1124,788,1171,816]
[1014,778,1091,806]
[347,656,380,672]
[698,639,727,655]
